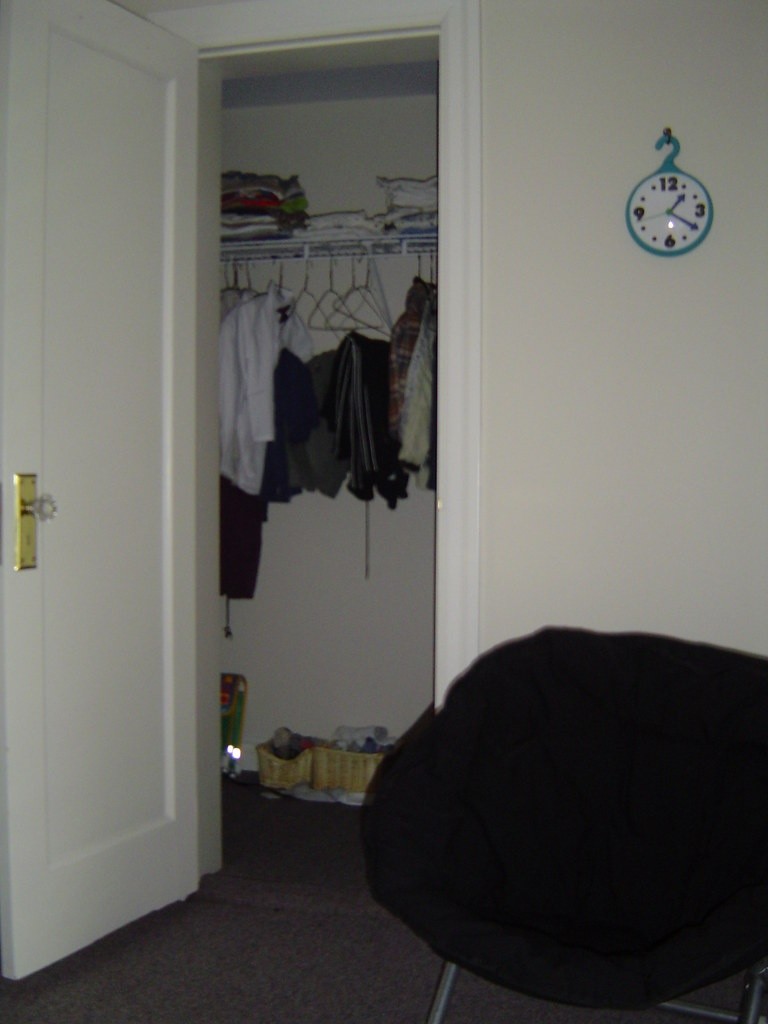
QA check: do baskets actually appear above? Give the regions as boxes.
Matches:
[312,740,387,792]
[255,735,327,789]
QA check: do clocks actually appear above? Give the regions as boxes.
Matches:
[624,168,714,255]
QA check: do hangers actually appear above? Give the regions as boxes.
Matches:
[220,248,439,337]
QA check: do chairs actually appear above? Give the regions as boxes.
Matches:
[350,624,768,1024]
